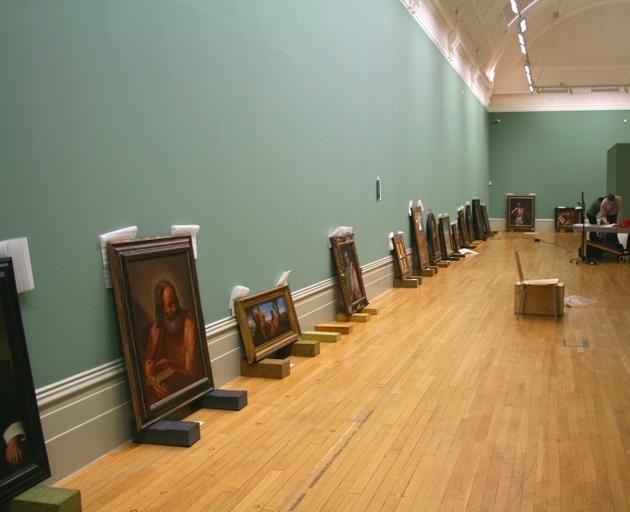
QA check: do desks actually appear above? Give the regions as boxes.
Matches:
[572,222,630,265]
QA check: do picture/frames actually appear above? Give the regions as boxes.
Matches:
[0,254,51,507]
[330,234,369,316]
[234,284,302,365]
[553,207,579,228]
[410,200,493,270]
[105,235,214,433]
[391,235,412,278]
[505,195,534,230]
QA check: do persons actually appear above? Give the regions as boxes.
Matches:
[598,193,623,245]
[0,362,32,479]
[141,279,204,406]
[511,202,527,225]
[586,197,604,241]
[250,305,280,338]
